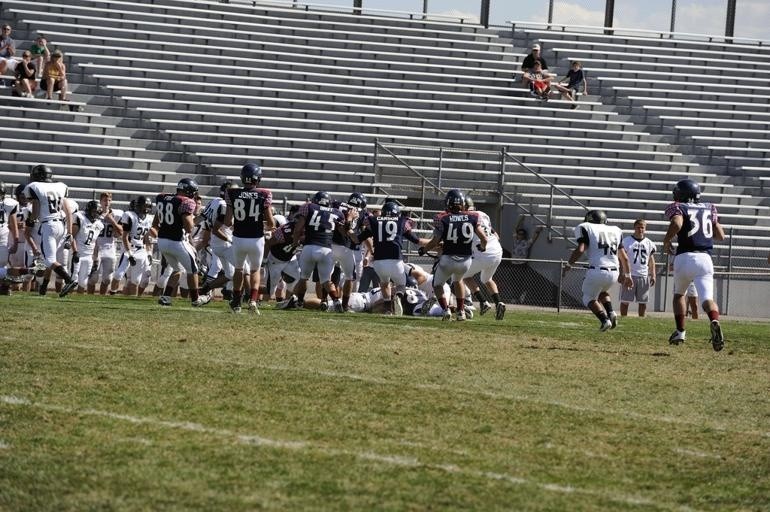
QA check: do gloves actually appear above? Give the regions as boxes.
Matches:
[562,263,570,277]
[128,256,136,266]
[26,217,34,227]
[623,272,634,290]
[64,241,79,263]
[418,247,427,256]
[476,244,486,251]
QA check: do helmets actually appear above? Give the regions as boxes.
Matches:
[445,189,475,211]
[85,201,103,223]
[312,191,400,217]
[585,210,607,224]
[133,196,152,215]
[220,163,262,200]
[0,165,53,195]
[176,178,198,199]
[673,179,701,203]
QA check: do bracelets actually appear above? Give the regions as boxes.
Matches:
[566,262,571,267]
[625,271,631,278]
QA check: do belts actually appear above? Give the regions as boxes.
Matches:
[583,266,617,270]
[42,219,62,223]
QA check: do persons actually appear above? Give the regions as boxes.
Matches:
[668,255,699,319]
[509,214,544,304]
[0,163,506,321]
[561,210,634,331]
[555,62,588,110]
[618,219,657,317]
[664,179,727,352]
[524,60,554,101]
[522,45,548,91]
[0,24,69,107]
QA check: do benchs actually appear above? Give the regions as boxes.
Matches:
[1,0,770,275]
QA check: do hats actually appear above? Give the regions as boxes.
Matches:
[532,44,540,50]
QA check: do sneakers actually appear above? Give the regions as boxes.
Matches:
[320,298,356,314]
[158,296,172,306]
[229,300,261,317]
[191,294,211,307]
[59,279,78,297]
[421,297,436,314]
[710,319,724,352]
[279,297,304,309]
[393,295,404,317]
[669,329,686,345]
[442,300,506,321]
[599,311,617,331]
